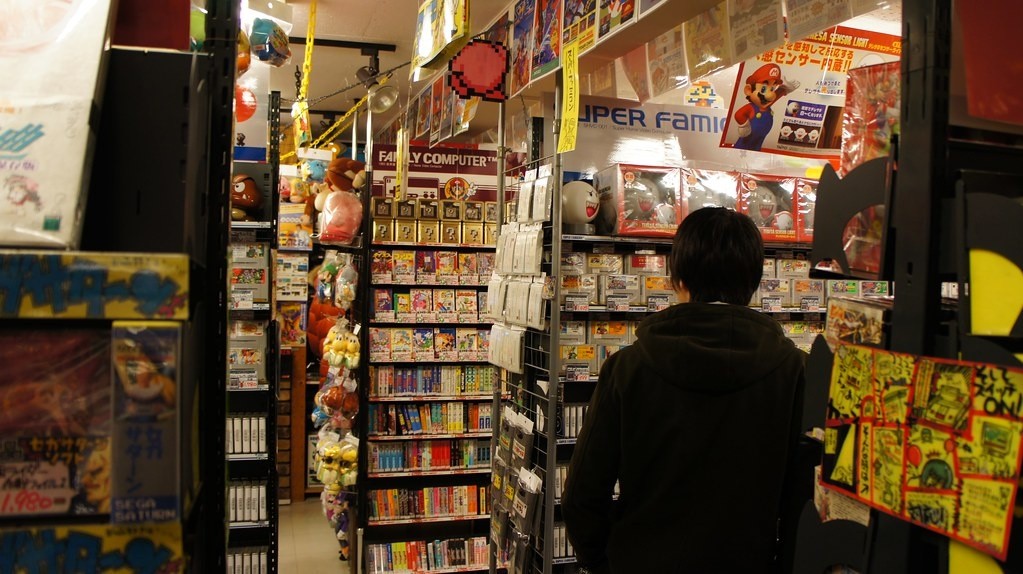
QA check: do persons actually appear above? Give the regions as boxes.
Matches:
[560,205,812,574]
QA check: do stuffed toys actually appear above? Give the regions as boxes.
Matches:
[308,156,365,561]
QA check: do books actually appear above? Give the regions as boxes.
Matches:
[365,250,496,574]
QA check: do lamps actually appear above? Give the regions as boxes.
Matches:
[356,65,400,114]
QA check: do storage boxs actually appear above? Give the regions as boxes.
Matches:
[307,430,328,488]
[0,250,191,574]
[366,193,519,250]
[553,160,896,377]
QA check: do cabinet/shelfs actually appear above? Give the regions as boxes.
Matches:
[355,170,497,544]
[221,91,282,574]
[489,118,970,574]
